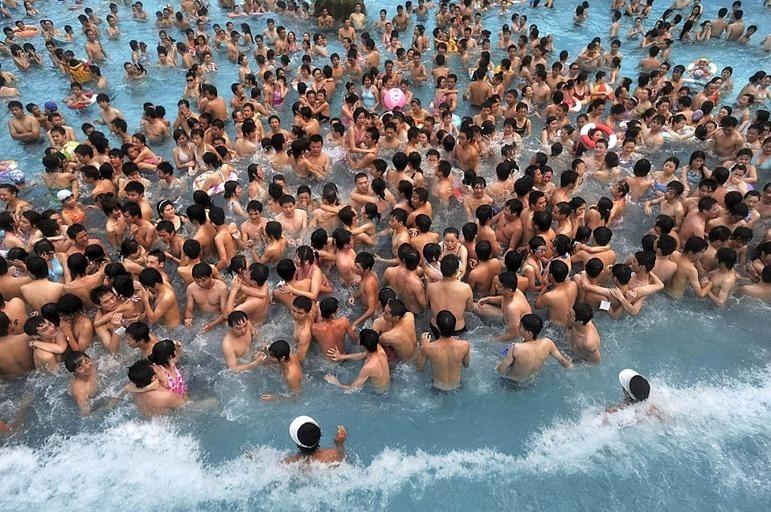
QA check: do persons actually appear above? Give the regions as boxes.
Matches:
[680,198,720,248]
[24,102,49,130]
[621,159,656,201]
[300,107,319,141]
[391,151,425,184]
[129,133,162,168]
[325,75,465,159]
[154,162,185,199]
[582,252,664,316]
[278,245,328,299]
[349,3,367,34]
[518,191,546,236]
[709,7,731,39]
[570,0,658,25]
[425,255,474,336]
[0,313,35,380]
[724,165,748,196]
[324,329,391,403]
[578,258,636,320]
[483,162,512,205]
[684,4,700,29]
[750,242,770,274]
[682,21,695,46]
[725,228,752,263]
[523,236,548,290]
[61,253,109,312]
[741,123,762,156]
[696,226,732,280]
[477,120,502,164]
[528,212,556,254]
[305,135,333,181]
[0,0,42,58]
[583,141,608,175]
[477,272,532,341]
[688,180,715,206]
[99,197,129,250]
[281,416,347,464]
[16,212,43,255]
[0,295,28,335]
[0,77,18,98]
[106,150,132,175]
[369,290,406,370]
[193,152,237,196]
[724,10,746,40]
[308,190,345,241]
[644,115,665,155]
[636,43,663,71]
[721,192,742,225]
[593,152,623,186]
[73,144,103,178]
[749,137,771,172]
[375,10,391,39]
[85,245,112,273]
[257,340,305,404]
[372,209,409,263]
[500,314,570,391]
[223,312,266,373]
[224,1,344,106]
[123,182,155,225]
[641,216,677,253]
[740,265,770,303]
[249,221,290,266]
[344,201,379,248]
[266,133,294,172]
[566,198,585,241]
[420,242,443,280]
[405,189,434,228]
[171,129,198,169]
[705,205,746,241]
[694,19,712,43]
[20,257,65,317]
[125,363,191,419]
[495,252,529,297]
[466,243,503,302]
[10,43,28,70]
[57,295,95,353]
[467,1,628,152]
[493,200,524,252]
[397,250,430,315]
[379,300,418,364]
[350,173,370,207]
[737,25,757,45]
[121,204,164,256]
[52,126,81,170]
[137,268,180,332]
[120,144,155,173]
[433,162,458,212]
[122,1,227,128]
[712,115,742,160]
[0,211,31,251]
[546,236,572,281]
[622,126,641,150]
[183,263,227,335]
[724,149,758,188]
[185,205,217,263]
[0,185,34,224]
[615,138,641,172]
[221,181,247,220]
[144,252,169,286]
[87,289,130,355]
[640,7,684,57]
[229,266,272,325]
[272,196,308,246]
[45,114,75,150]
[736,192,761,229]
[708,168,730,203]
[77,1,126,40]
[28,302,69,356]
[289,138,316,180]
[7,100,43,146]
[251,138,277,167]
[0,256,30,302]
[496,120,523,162]
[177,240,221,282]
[350,179,391,215]
[21,41,43,66]
[566,305,599,368]
[394,5,409,31]
[311,299,358,368]
[462,177,492,222]
[605,180,629,220]
[611,55,771,117]
[81,28,107,64]
[42,101,60,128]
[64,224,99,261]
[90,276,149,326]
[206,208,239,273]
[474,205,500,253]
[41,156,75,198]
[22,317,60,377]
[649,157,679,192]
[411,214,438,263]
[586,198,612,232]
[80,165,116,206]
[701,250,738,306]
[261,116,293,143]
[549,171,581,212]
[604,370,660,413]
[391,181,417,221]
[551,201,572,242]
[63,82,91,113]
[335,31,420,82]
[93,93,122,130]
[462,222,479,255]
[533,261,578,322]
[119,240,146,276]
[38,18,76,68]
[231,201,269,249]
[225,254,258,305]
[645,180,685,228]
[190,129,222,166]
[454,129,477,178]
[103,263,141,302]
[411,0,475,78]
[567,140,584,160]
[569,159,587,193]
[350,251,382,325]
[370,159,387,187]
[125,323,159,358]
[235,123,257,161]
[337,207,370,250]
[32,240,70,284]
[117,163,152,197]
[420,311,470,396]
[506,152,557,212]
[158,220,188,267]
[682,153,710,198]
[156,201,191,239]
[270,260,334,314]
[56,189,87,231]
[64,49,108,91]
[626,18,643,41]
[510,102,531,142]
[245,162,270,204]
[664,235,712,299]
[64,351,108,413]
[39,219,72,254]
[438,228,468,280]
[573,227,618,286]
[647,236,677,287]
[125,341,187,394]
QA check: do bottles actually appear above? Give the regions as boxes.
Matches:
[10,170,25,184]
[56,190,74,202]
[619,369,651,401]
[45,101,58,111]
[289,415,322,449]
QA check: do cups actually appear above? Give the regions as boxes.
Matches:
[531,247,548,252]
[65,194,74,199]
[187,79,193,82]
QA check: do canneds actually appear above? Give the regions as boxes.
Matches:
[66,90,98,109]
[293,76,316,92]
[580,123,617,150]
[686,59,716,77]
[193,170,237,196]
[12,24,39,38]
[0,160,18,176]
[448,113,461,133]
[561,96,582,113]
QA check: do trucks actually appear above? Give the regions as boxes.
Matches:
[384,87,405,110]
